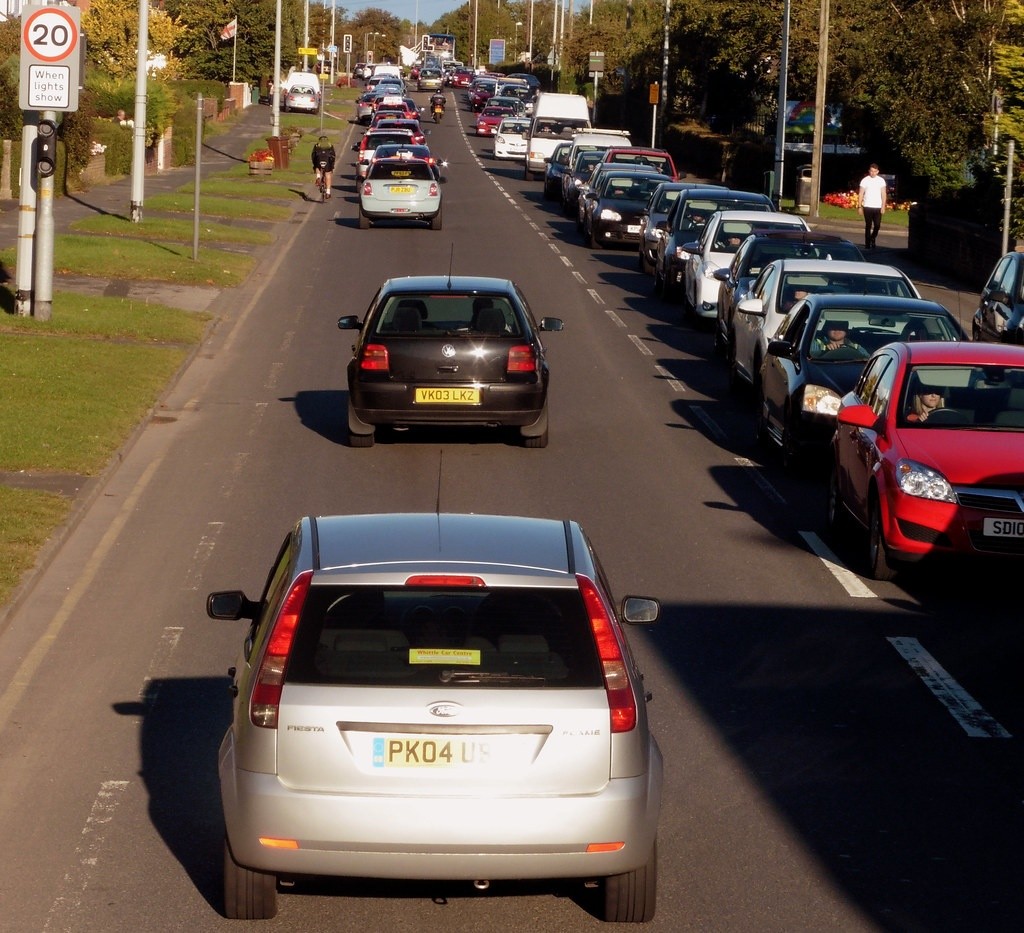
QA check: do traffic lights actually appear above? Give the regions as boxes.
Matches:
[343,35,352,53]
[36,120,59,178]
[429,45,434,51]
[421,34,429,52]
[367,51,373,64]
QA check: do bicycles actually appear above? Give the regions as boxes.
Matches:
[312,159,334,203]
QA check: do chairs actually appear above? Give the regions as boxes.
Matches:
[470,297,495,327]
[372,140,383,148]
[561,126,573,136]
[396,299,429,319]
[899,320,929,343]
[992,387,1024,425]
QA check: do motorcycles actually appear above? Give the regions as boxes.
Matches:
[429,96,447,124]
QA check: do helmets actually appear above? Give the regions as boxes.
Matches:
[436,89,441,94]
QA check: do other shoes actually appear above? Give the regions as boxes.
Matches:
[326,194,331,199]
[315,178,322,186]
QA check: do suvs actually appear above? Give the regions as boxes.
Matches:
[971,252,1024,348]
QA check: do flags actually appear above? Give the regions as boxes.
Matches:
[219,19,235,41]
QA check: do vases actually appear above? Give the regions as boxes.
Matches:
[248,162,274,175]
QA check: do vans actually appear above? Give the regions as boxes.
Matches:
[490,117,532,161]
[521,92,594,180]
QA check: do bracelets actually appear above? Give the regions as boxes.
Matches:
[858,207,861,208]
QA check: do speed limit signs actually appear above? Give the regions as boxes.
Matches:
[24,8,79,61]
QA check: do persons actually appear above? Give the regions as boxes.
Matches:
[857,163,887,251]
[112,109,125,123]
[312,135,336,199]
[726,233,741,247]
[783,284,816,312]
[430,88,446,118]
[688,210,707,227]
[906,375,945,422]
[815,319,849,351]
[458,297,511,333]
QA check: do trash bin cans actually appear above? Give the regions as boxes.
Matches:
[251,87,259,104]
[795,164,812,205]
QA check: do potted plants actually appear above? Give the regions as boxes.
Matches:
[281,126,303,153]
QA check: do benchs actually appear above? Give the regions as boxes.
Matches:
[293,89,311,93]
[319,590,579,673]
[392,307,508,331]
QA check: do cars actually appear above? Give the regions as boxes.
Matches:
[712,229,869,371]
[638,182,732,276]
[759,291,972,484]
[355,159,448,231]
[283,84,320,114]
[474,106,518,137]
[728,256,925,424]
[681,208,821,334]
[209,510,667,919]
[352,63,366,80]
[316,60,333,75]
[824,342,1024,582]
[337,274,564,450]
[655,189,780,304]
[416,67,446,93]
[260,51,689,251]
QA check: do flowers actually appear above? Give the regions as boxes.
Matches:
[246,148,275,163]
